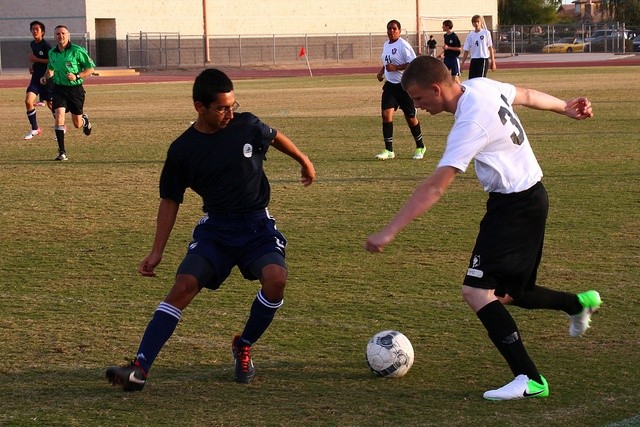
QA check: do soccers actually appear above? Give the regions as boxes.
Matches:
[365,330,415,380]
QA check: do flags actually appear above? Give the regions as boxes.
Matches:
[297,46,306,58]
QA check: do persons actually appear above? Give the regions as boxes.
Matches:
[427,35,437,58]
[375,20,426,161]
[40,25,95,161]
[105,68,316,392]
[365,55,603,401]
[22,20,68,140]
[436,20,462,85]
[460,14,496,79]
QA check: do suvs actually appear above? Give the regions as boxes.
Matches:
[585,29,628,43]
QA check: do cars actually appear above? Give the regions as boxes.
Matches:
[630,35,640,53]
[543,37,589,53]
[501,31,521,42]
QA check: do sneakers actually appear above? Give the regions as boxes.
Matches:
[230,333,257,385]
[23,127,42,139]
[482,374,550,403]
[54,150,69,162]
[54,126,67,140]
[82,114,92,137]
[412,144,426,160]
[567,289,604,341]
[104,360,149,393]
[375,149,395,161]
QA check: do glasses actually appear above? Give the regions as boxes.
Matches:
[208,100,240,116]
